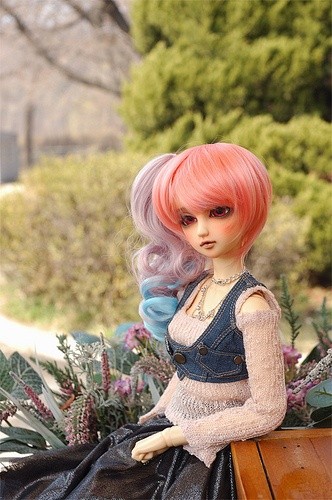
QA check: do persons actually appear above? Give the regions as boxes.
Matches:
[1,142,288,499]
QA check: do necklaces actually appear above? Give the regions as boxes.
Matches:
[191,270,247,321]
